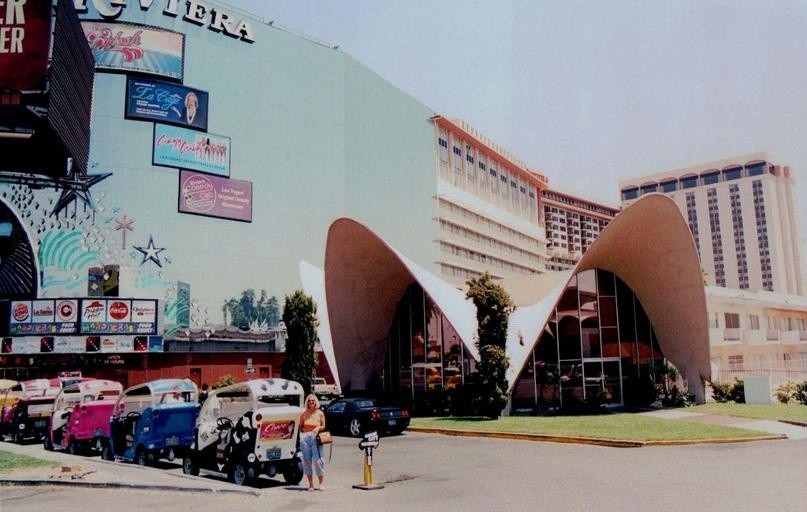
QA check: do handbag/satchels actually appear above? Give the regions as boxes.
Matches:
[317,432,331,445]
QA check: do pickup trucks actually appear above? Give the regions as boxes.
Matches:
[311,376,342,400]
[319,395,411,437]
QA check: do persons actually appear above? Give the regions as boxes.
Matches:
[178,92,206,128]
[299,393,326,491]
[162,387,185,404]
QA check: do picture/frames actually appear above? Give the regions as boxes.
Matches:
[82,16,255,224]
